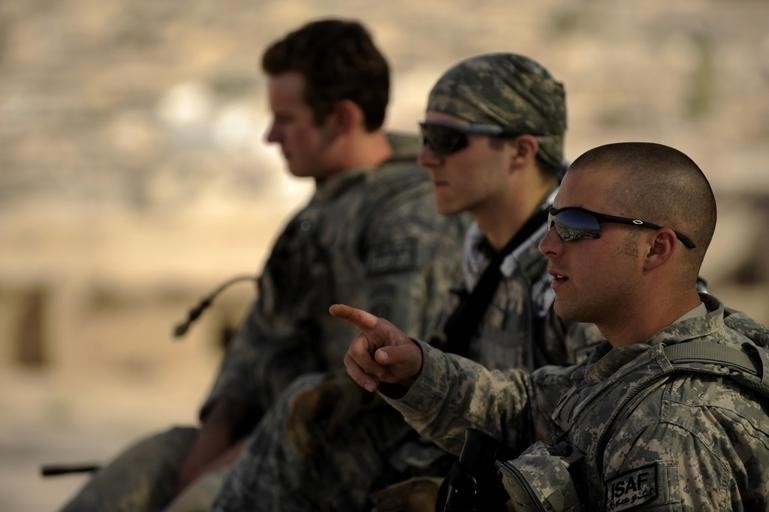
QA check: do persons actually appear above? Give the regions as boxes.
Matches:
[207,50,576,511]
[44,18,479,510]
[327,141,768,511]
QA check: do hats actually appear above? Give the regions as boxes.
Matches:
[427,51,566,164]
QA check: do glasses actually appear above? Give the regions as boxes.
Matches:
[546,203,698,251]
[418,119,514,156]
